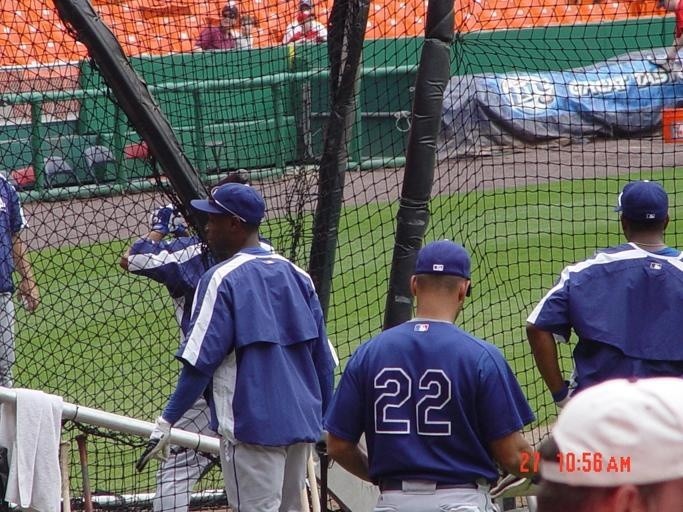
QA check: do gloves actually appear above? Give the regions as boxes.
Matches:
[151,203,188,235]
[136,417,171,472]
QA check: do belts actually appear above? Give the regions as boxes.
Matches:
[380,479,479,490]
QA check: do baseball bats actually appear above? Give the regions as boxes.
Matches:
[59,433,93,512]
[174,168,248,216]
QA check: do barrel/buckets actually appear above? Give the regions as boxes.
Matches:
[661,107,683,143]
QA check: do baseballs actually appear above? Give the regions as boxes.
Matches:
[21,295,30,309]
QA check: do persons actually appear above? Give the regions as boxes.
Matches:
[526,180,683,408]
[136,183,339,512]
[537,375,683,512]
[192,4,253,53]
[120,203,276,512]
[282,0,328,45]
[0,174,40,388]
[322,239,541,512]
[651,0,683,73]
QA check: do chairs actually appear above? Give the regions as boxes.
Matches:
[0,0,675,71]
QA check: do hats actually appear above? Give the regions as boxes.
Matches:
[415,239,471,297]
[614,182,668,221]
[538,375,682,487]
[190,182,265,225]
